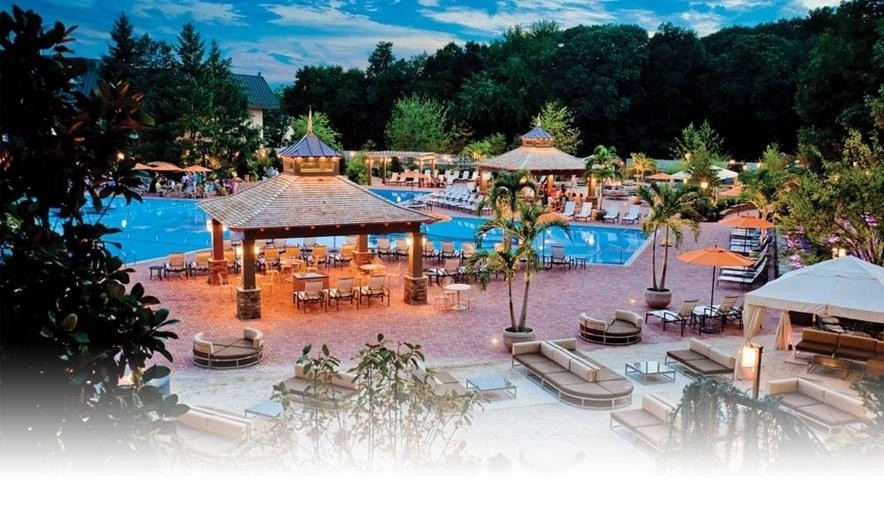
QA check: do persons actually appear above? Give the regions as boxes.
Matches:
[150,173,240,196]
[533,182,587,215]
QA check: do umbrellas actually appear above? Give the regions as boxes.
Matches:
[674,242,756,317]
[644,172,675,187]
[718,186,747,216]
[124,160,212,175]
[721,212,776,252]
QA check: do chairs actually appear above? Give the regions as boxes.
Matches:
[645,228,771,337]
[389,169,641,225]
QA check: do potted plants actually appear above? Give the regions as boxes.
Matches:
[636,181,718,308]
[583,145,626,220]
[467,170,572,351]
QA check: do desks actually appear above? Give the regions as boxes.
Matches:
[149,265,164,280]
[575,257,586,269]
